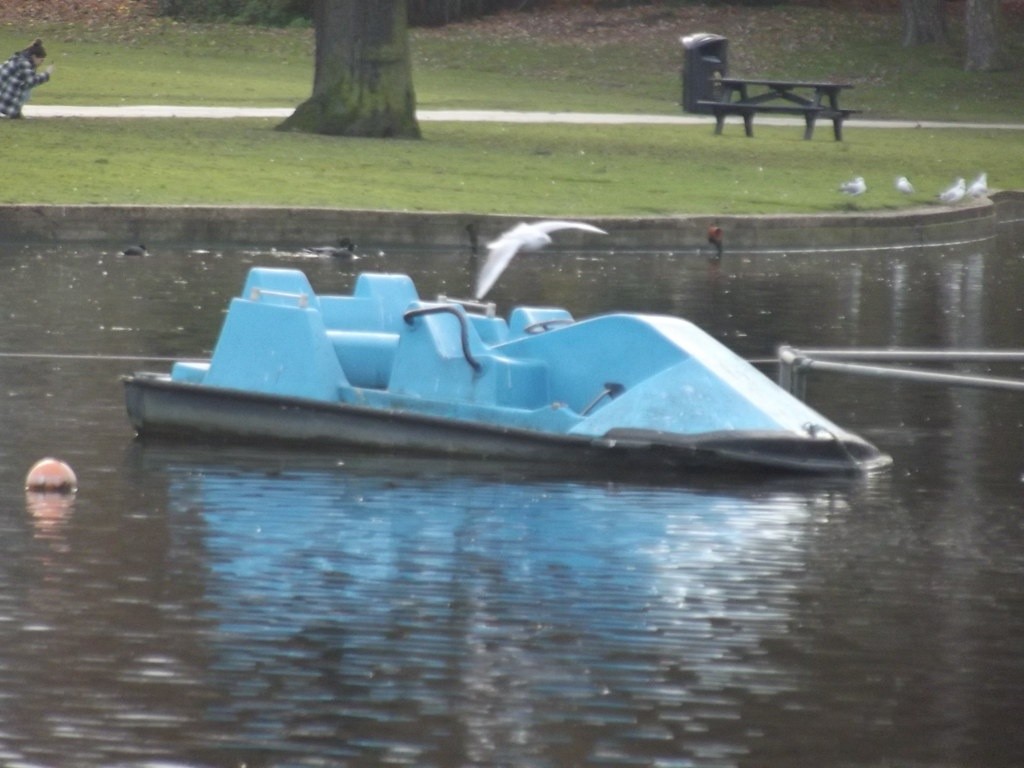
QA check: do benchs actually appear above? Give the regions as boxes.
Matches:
[697,76,864,142]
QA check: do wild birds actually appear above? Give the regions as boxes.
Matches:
[474,218,608,303]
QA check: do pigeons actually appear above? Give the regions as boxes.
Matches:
[840,177,867,197]
[936,179,965,205]
[967,173,989,199]
[896,176,915,195]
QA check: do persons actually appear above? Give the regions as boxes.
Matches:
[0,38,57,118]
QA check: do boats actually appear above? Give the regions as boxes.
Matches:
[113,261,897,479]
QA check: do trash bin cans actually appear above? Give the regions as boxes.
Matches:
[680,32,727,115]
